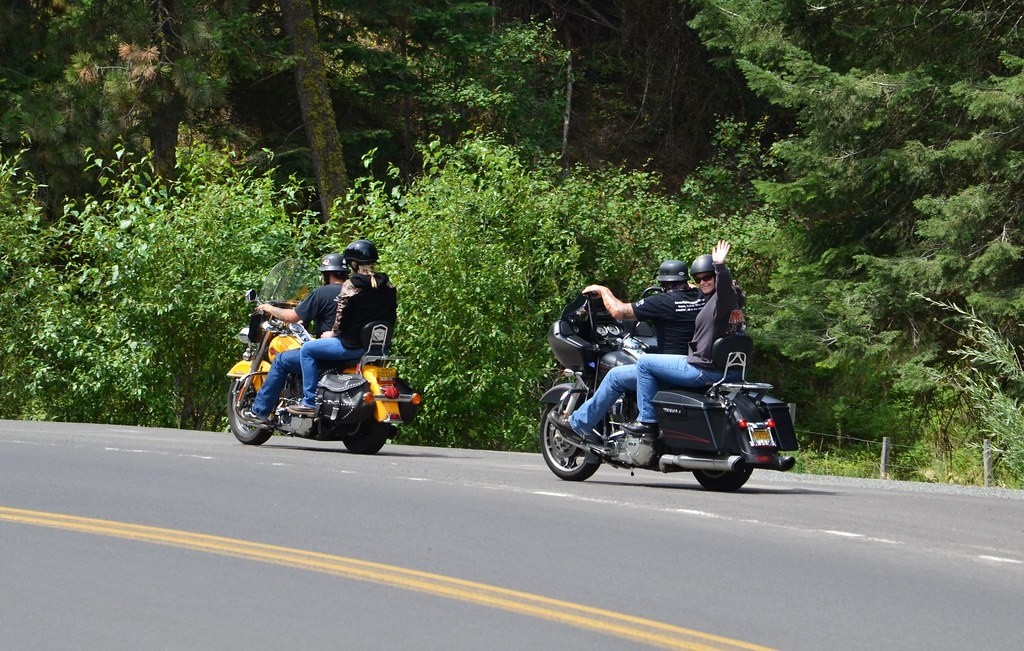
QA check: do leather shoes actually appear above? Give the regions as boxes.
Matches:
[620,422,659,436]
[547,411,585,439]
[287,404,316,416]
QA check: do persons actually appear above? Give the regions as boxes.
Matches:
[549,259,710,440]
[619,240,747,437]
[288,240,397,418]
[241,254,352,424]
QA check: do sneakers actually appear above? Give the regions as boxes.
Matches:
[241,408,267,422]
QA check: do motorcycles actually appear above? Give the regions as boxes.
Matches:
[539,279,798,492]
[226,259,421,454]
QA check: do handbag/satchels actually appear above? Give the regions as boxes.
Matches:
[313,373,375,424]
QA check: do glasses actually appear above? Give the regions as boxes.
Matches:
[694,274,713,284]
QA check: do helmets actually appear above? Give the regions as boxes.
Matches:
[656,259,691,281]
[342,240,379,262]
[690,254,715,274]
[318,253,351,273]
